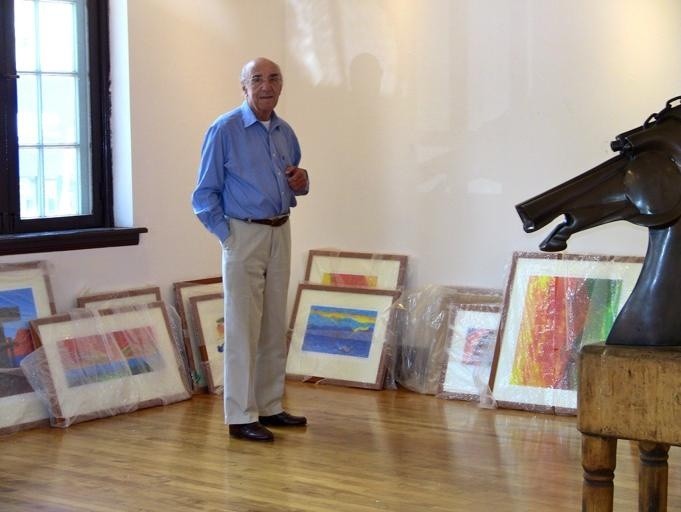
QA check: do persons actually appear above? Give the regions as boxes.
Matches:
[192,58,309,443]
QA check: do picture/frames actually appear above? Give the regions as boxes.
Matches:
[284,281,402,391]
[422,288,509,403]
[28,300,192,428]
[304,251,408,389]
[171,276,226,393]
[189,293,225,395]
[486,252,643,418]
[78,287,162,311]
[1,260,57,434]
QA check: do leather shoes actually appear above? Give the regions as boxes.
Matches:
[229,421,274,442]
[257,411,308,428]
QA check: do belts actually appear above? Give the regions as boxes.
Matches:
[231,215,289,228]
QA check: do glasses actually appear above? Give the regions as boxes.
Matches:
[247,77,282,87]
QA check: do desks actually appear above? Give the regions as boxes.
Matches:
[577,340,680,512]
